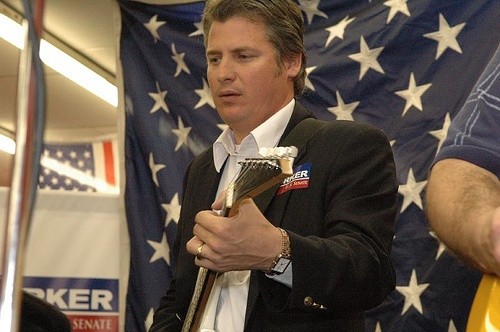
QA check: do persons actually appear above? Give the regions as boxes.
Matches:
[426,43,500,276]
[148,0,399,331]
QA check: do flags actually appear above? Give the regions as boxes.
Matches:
[112,0,500,332]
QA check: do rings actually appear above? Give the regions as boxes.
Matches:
[195,242,205,259]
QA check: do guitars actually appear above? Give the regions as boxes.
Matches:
[178,144,298,332]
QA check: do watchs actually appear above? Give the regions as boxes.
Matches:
[265,226,292,277]
